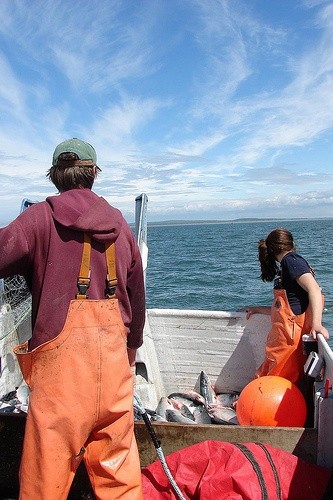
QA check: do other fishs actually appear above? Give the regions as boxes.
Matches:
[155,370,238,424]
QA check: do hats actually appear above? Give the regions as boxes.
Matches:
[52,136,104,173]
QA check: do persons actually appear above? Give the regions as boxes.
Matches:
[0,138,148,500]
[233,229,329,410]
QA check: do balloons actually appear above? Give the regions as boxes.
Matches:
[236,377,307,428]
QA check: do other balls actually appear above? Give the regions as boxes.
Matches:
[234,374,308,428]
